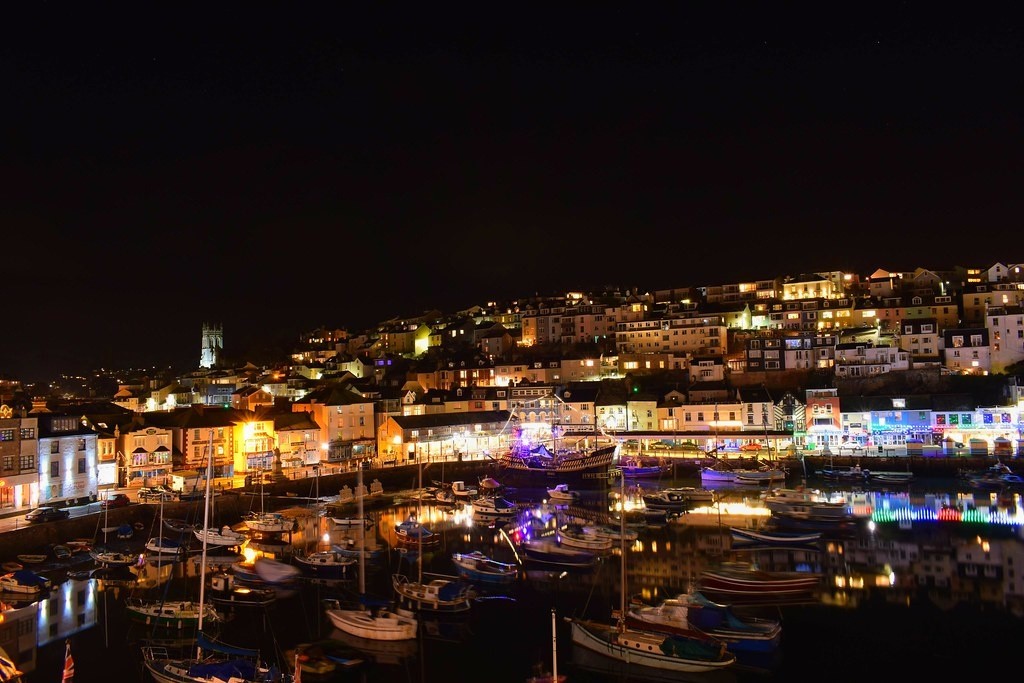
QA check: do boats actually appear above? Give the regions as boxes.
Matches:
[4,417,1018,681]
